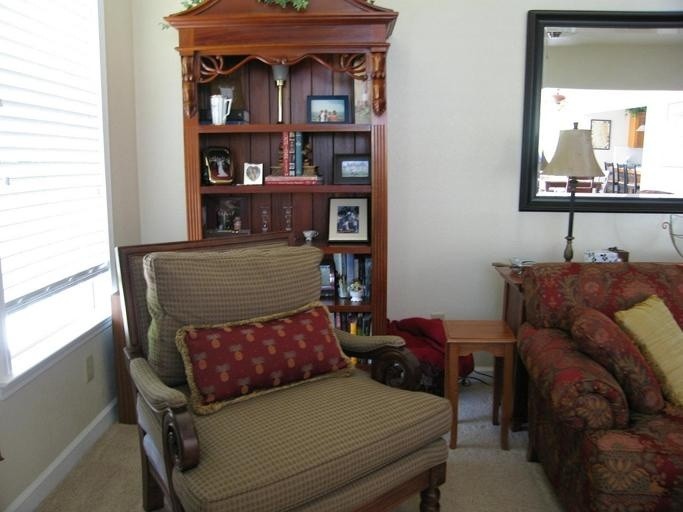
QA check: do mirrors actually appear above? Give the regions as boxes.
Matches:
[518,9,683,214]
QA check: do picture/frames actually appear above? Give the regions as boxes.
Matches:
[332,153,371,185]
[307,94,352,124]
[326,197,370,245]
[590,119,611,150]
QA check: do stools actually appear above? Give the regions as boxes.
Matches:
[441,319,516,450]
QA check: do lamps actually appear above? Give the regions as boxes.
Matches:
[539,122,605,263]
[551,88,566,105]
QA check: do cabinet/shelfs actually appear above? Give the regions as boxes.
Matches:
[493,260,527,433]
[627,112,646,148]
[163,0,401,342]
[109,291,137,424]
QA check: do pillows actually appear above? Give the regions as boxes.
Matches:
[568,305,664,414]
[143,243,325,412]
[177,300,358,416]
[614,293,683,406]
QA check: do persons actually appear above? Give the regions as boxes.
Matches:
[216,205,237,231]
[215,154,229,177]
[337,207,358,233]
[317,110,337,122]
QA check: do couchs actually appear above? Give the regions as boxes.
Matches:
[516,263,683,512]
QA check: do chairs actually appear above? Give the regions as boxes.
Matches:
[114,233,448,512]
[604,162,639,193]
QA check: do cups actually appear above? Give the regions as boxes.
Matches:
[301,230,319,241]
[209,94,233,125]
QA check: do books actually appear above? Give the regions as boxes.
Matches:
[264,174,322,182]
[282,131,288,176]
[319,253,371,368]
[295,132,304,176]
[264,180,323,185]
[287,132,296,176]
